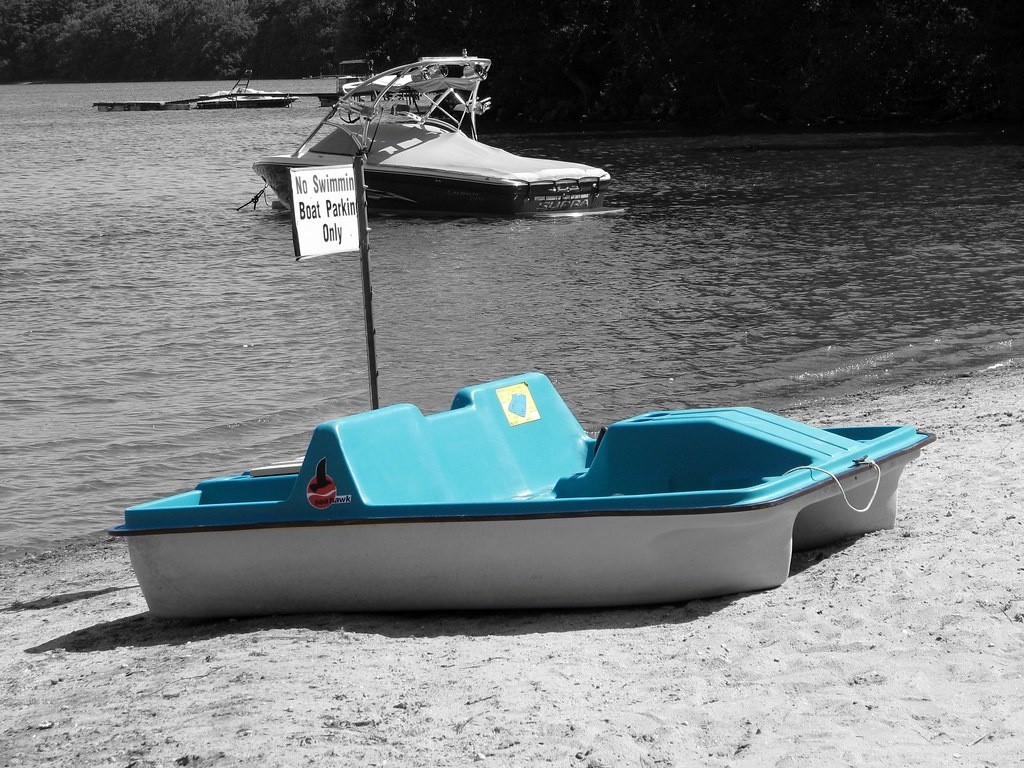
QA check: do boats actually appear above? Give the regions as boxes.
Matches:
[247,47,627,221]
[232,57,418,108]
[106,149,938,626]
[90,66,302,113]
